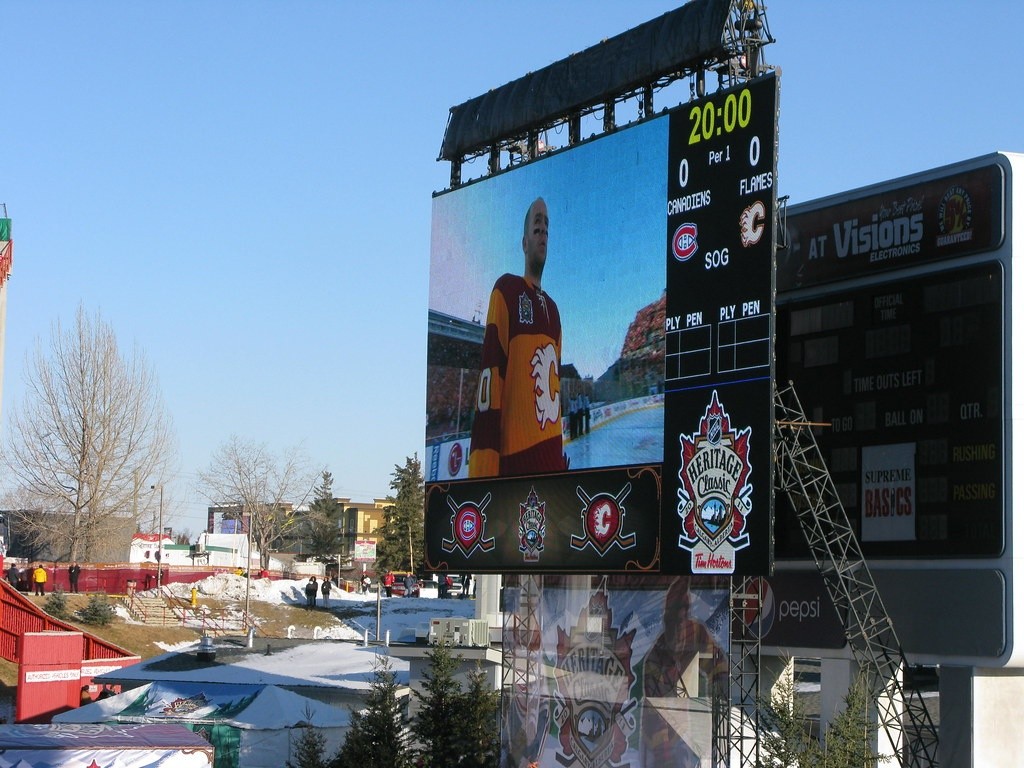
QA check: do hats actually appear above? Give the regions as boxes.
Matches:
[11,563,16,567]
[308,580,314,584]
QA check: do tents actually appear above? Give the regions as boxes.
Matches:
[0,681,357,768]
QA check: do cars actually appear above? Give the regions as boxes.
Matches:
[388,571,420,599]
[446,577,464,598]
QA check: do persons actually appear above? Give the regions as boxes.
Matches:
[436,573,449,600]
[469,197,566,478]
[404,572,413,597]
[384,569,395,597]
[459,574,472,600]
[8,563,21,588]
[306,576,318,605]
[68,561,80,593]
[322,577,331,607]
[234,567,243,576]
[81,685,92,706]
[482,733,497,768]
[33,565,46,596]
[107,685,116,698]
[258,566,269,578]
[361,575,371,594]
[569,393,590,440]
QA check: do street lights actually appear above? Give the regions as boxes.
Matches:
[151,484,164,588]
[239,511,252,630]
[334,554,340,589]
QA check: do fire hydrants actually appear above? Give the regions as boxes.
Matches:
[191,588,198,606]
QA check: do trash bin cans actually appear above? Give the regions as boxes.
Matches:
[127,578,137,596]
